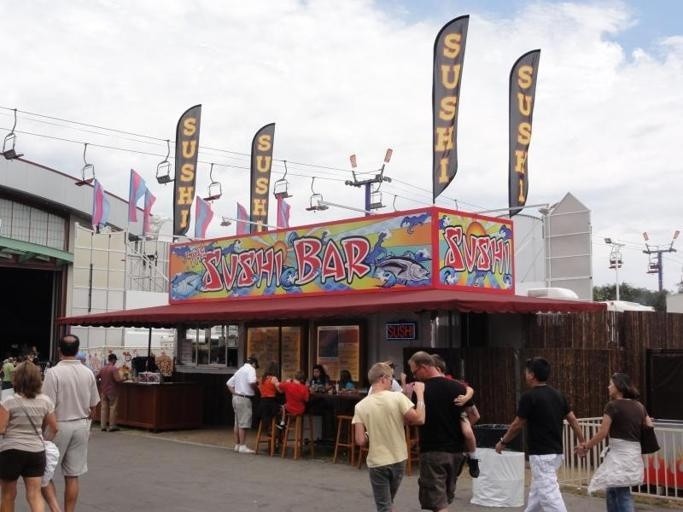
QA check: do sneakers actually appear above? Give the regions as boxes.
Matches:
[109,425,120,431]
[100,426,106,431]
[234,444,256,453]
[466,455,481,477]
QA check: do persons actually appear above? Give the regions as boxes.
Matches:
[575,373,654,512]
[352,351,481,512]
[0,335,100,512]
[95,354,128,432]
[495,358,588,512]
[226,355,354,453]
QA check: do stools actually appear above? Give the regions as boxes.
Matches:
[403,423,421,476]
[332,415,355,466]
[281,413,315,459]
[255,412,284,457]
[358,447,368,469]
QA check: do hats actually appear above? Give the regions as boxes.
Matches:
[245,357,259,369]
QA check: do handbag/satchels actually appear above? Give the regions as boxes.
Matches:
[41,441,60,488]
[641,425,661,454]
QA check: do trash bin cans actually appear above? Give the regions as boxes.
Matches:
[470,424,526,508]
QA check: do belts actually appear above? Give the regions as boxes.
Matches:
[240,395,250,398]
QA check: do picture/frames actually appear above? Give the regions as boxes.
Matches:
[384,320,418,340]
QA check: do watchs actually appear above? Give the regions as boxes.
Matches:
[583,445,588,452]
[500,437,507,446]
